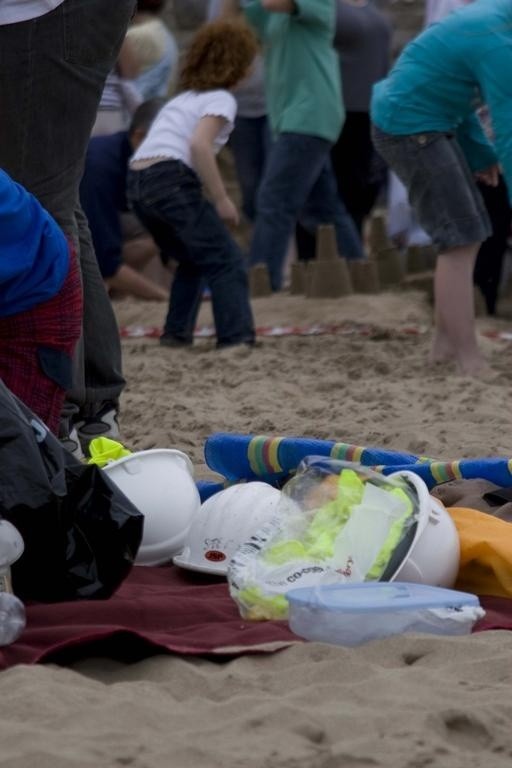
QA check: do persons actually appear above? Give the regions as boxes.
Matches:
[0,169,83,440]
[77,1,398,353]
[370,0,511,379]
[390,0,512,316]
[0,0,140,462]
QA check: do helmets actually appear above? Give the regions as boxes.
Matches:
[100,447,202,570]
[172,481,303,577]
[387,470,461,589]
